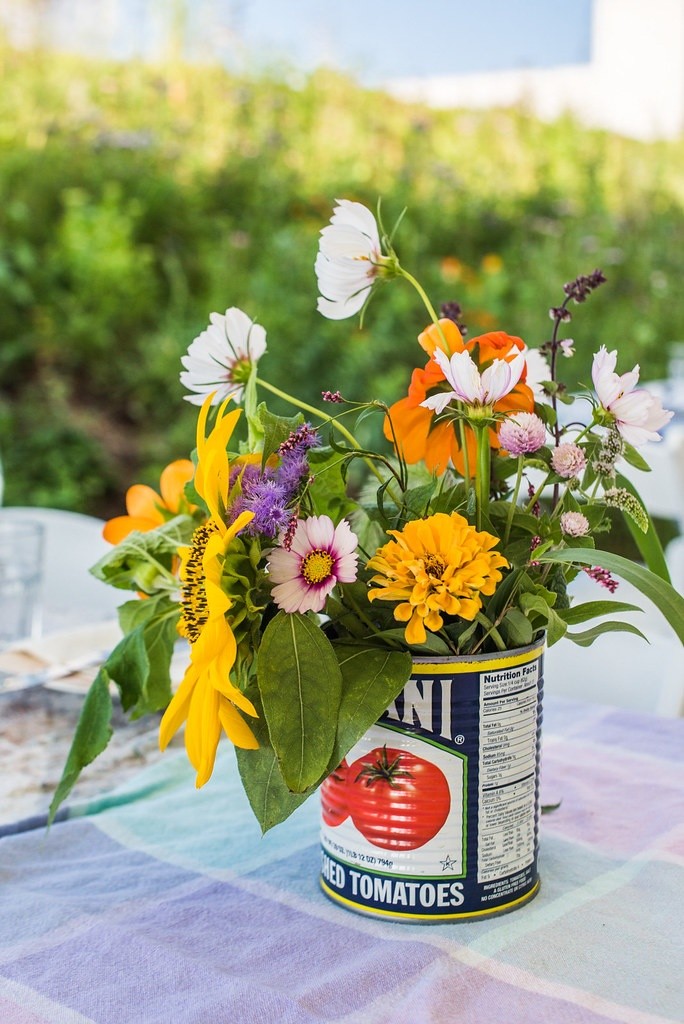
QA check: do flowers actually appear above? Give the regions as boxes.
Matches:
[45,197,684,834]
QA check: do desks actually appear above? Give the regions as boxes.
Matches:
[0,502,684,1024]
